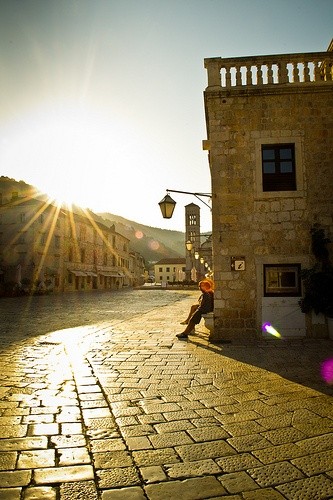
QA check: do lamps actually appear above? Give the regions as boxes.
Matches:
[185,234,212,272]
[159,189,213,219]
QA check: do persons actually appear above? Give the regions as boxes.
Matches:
[176,279,214,338]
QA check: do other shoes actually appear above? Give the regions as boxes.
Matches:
[176,332,188,338]
[180,321,188,325]
[188,331,195,336]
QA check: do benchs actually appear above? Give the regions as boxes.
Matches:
[201,312,214,331]
